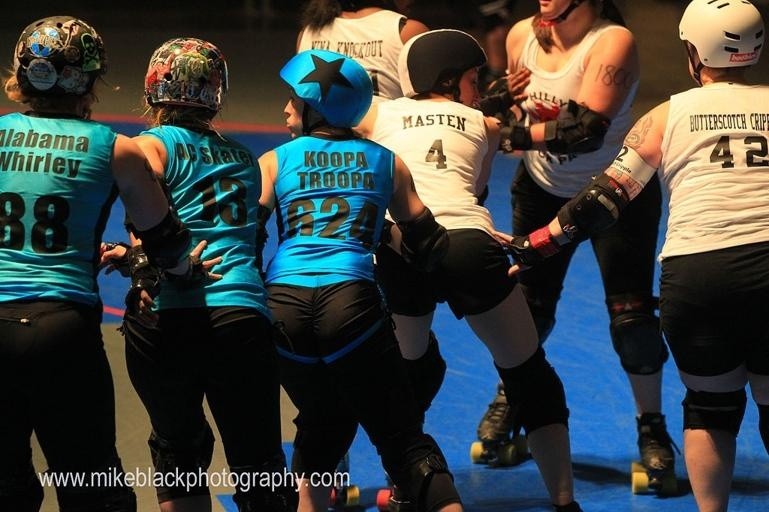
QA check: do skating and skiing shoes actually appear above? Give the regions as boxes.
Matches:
[625,410,685,500]
[466,382,533,467]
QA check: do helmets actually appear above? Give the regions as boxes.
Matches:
[677,0,767,69]
[142,33,230,117]
[277,47,374,131]
[395,26,489,103]
[12,12,110,107]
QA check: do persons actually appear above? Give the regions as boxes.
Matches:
[124,38,300,512]
[257,48,465,511]
[0,16,222,512]
[296,0,431,99]
[373,28,586,512]
[485,0,769,512]
[476,0,676,474]
